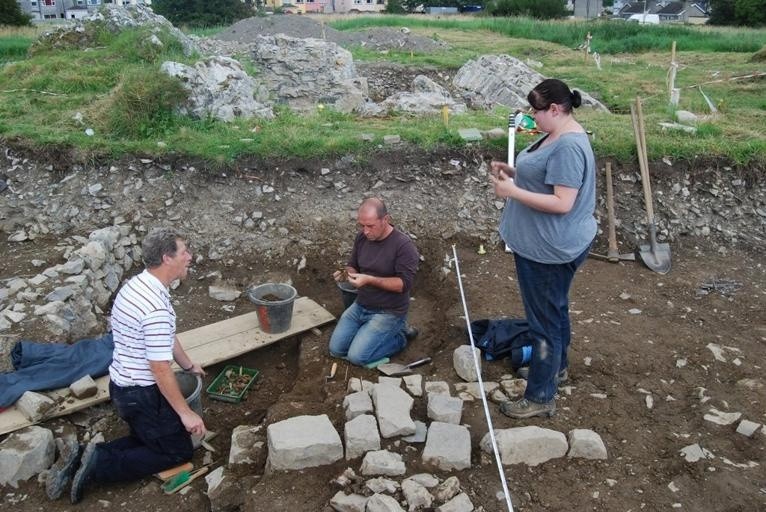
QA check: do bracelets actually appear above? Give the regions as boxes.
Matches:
[181,364,195,372]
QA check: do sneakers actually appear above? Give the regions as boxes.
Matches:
[406,326,420,339]
[71,441,99,504]
[500,396,557,417]
[518,366,568,383]
[46,442,77,501]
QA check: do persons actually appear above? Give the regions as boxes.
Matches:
[485,77,601,421]
[325,194,422,370]
[42,225,209,504]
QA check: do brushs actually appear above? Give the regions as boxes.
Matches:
[160,467,209,495]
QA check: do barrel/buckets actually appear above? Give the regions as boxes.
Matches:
[172,370,206,421]
[336,280,360,308]
[247,283,296,335]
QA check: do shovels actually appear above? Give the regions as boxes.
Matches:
[323,362,337,384]
[377,356,432,376]
[192,432,216,452]
[629,96,672,276]
